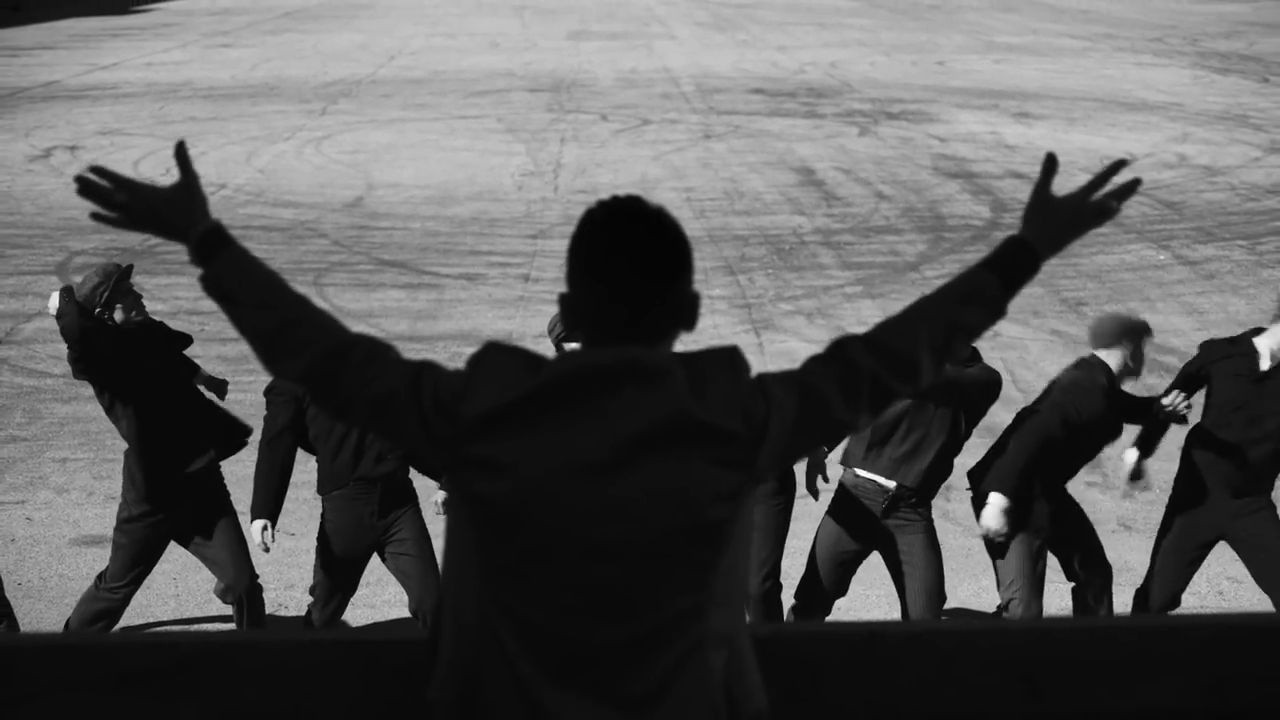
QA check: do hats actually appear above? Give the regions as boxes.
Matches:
[1087,311,1154,349]
[77,261,135,312]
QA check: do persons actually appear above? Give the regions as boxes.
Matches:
[48,262,269,634]
[1123,298,1280,615]
[71,138,1141,720]
[433,309,796,624]
[251,376,448,622]
[967,313,1152,619]
[795,300,1010,621]
